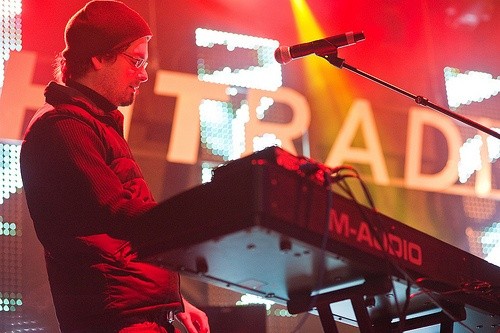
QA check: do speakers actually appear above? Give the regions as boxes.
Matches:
[205,303,266,333]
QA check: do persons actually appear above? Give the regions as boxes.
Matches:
[20,0,210,332]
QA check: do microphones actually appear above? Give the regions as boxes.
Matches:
[273,31,365,68]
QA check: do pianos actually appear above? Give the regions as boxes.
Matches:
[125,168,500,333]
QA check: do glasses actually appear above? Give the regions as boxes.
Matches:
[120,51,149,69]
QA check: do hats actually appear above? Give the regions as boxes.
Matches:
[61,0,154,62]
